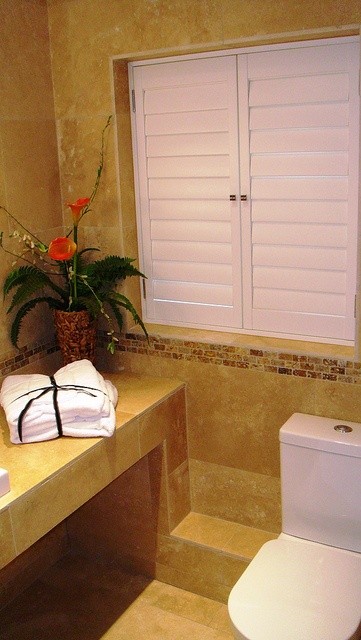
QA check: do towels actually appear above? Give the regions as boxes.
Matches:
[0,359,118,443]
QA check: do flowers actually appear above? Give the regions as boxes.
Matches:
[0,113,153,355]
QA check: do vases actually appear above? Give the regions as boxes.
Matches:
[54,310,97,369]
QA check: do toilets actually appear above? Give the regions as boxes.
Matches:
[227,412,361,640]
